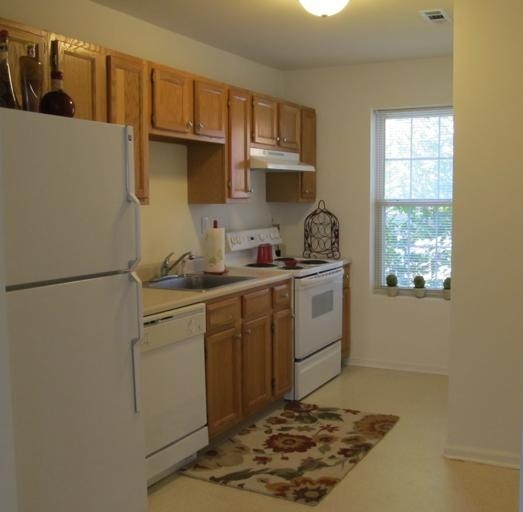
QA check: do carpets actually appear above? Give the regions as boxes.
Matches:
[178,400,400,507]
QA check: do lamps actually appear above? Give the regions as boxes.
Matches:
[299,0,349,18]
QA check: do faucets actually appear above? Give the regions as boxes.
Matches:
[168,250,196,274]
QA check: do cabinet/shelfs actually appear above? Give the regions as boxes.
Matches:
[205,284,272,441]
[0,14,50,114]
[51,32,149,206]
[271,278,292,403]
[252,90,301,155]
[147,60,227,147]
[188,71,251,204]
[266,105,316,202]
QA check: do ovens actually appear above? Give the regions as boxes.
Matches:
[294,267,345,402]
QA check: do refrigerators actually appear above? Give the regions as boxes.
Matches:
[0,106,147,511]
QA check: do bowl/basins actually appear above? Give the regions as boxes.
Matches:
[284,260,298,268]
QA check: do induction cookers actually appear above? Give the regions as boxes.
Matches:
[228,256,343,274]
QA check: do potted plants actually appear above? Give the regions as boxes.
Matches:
[443,277,452,300]
[385,272,398,297]
[412,276,426,299]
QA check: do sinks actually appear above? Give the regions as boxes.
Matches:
[142,273,258,293]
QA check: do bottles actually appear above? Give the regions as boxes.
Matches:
[0,29,20,109]
[41,70,75,117]
[20,44,44,112]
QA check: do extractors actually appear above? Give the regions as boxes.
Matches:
[250,149,316,172]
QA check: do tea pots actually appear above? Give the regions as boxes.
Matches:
[256,244,273,264]
